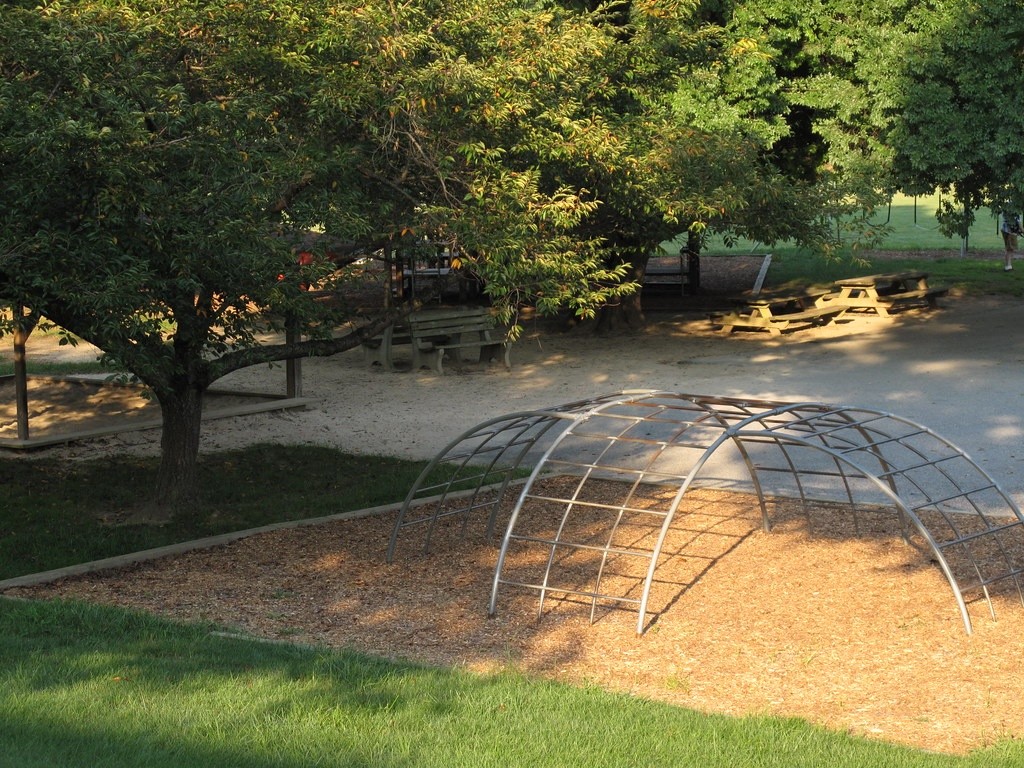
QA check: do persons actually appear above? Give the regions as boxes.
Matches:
[300,253,314,291]
[444,248,492,307]
[1001,199,1024,272]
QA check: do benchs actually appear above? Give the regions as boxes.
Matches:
[363,319,460,371]
[405,306,514,373]
[709,285,948,324]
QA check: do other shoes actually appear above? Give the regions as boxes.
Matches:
[1003,266,1016,272]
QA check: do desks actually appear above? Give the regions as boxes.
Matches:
[834,271,937,317]
[721,282,835,336]
